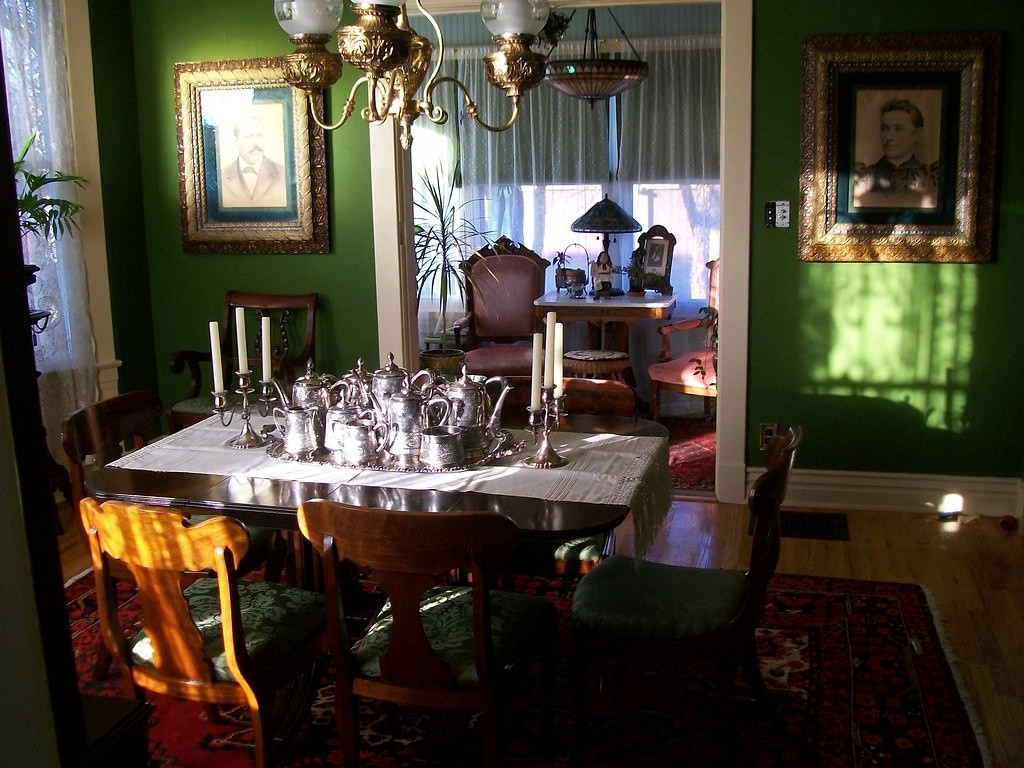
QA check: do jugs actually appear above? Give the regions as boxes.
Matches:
[273,406,320,462]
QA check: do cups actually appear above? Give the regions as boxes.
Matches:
[418,348,467,375]
[418,425,466,468]
[329,418,389,468]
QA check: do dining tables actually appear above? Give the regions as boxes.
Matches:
[86,398,668,747]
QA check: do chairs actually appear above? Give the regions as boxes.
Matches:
[650,254,719,430]
[452,233,552,428]
[77,498,324,768]
[568,425,799,768]
[167,293,314,440]
[61,388,290,672]
[296,497,558,768]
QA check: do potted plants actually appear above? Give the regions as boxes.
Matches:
[553,251,574,292]
[408,148,506,384]
[624,262,646,296]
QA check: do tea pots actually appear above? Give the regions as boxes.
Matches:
[270,352,515,468]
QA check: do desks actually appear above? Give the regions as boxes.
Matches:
[535,285,677,428]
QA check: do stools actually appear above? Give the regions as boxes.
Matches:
[563,349,629,388]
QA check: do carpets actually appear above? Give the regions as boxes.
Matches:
[748,509,851,542]
[50,548,980,768]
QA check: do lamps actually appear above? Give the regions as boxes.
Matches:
[274,0,550,151]
[210,304,278,450]
[522,309,568,467]
[570,192,642,295]
[544,0,649,109]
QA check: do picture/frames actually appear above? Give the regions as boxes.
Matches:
[632,225,677,296]
[797,28,995,266]
[172,55,331,256]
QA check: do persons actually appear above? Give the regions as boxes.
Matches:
[222,120,285,208]
[855,99,939,194]
[652,245,663,264]
[589,253,615,300]
